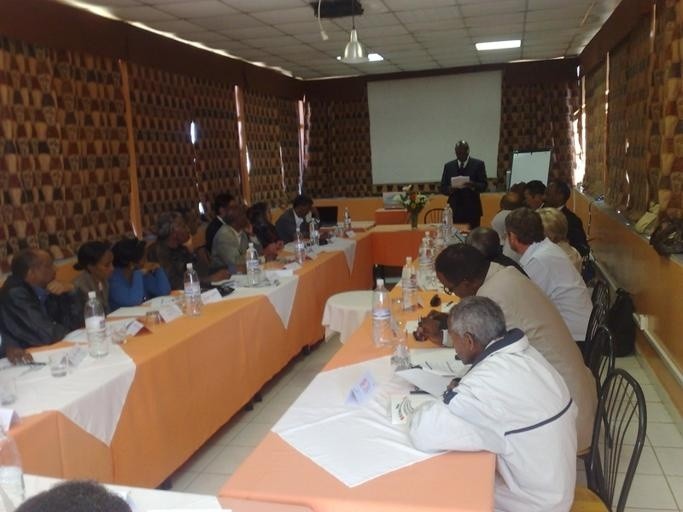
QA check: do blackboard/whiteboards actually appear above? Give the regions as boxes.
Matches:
[509,150,551,191]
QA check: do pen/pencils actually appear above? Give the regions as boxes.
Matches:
[410,391,429,394]
[26,363,46,365]
[447,301,453,306]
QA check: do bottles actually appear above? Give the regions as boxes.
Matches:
[293,206,352,266]
[83,291,108,359]
[400,256,418,313]
[417,203,452,279]
[245,242,261,289]
[371,278,392,348]
[182,262,203,315]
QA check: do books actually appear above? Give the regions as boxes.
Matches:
[63,318,132,344]
[389,392,434,423]
[106,304,156,317]
[396,367,452,400]
[450,175,470,192]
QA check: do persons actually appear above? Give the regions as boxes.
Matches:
[401,297,579,511]
[205,194,314,273]
[1,247,86,366]
[16,482,131,512]
[436,139,487,231]
[466,181,593,365]
[72,208,230,316]
[416,242,599,455]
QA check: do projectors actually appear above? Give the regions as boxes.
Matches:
[313,0,364,18]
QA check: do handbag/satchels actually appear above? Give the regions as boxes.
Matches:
[649,215,683,255]
[635,201,660,234]
[600,288,634,357]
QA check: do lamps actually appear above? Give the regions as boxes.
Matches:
[336,1,371,65]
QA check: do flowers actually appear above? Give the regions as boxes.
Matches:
[397,184,430,225]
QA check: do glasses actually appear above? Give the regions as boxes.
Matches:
[444,281,463,295]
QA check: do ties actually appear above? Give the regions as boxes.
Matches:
[460,161,464,168]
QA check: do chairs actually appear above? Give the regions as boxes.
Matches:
[1,208,652,512]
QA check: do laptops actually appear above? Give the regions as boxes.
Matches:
[312,206,338,227]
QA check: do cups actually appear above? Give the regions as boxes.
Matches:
[144,310,164,332]
[0,375,17,407]
[48,351,68,379]
[110,321,128,345]
[394,344,411,369]
